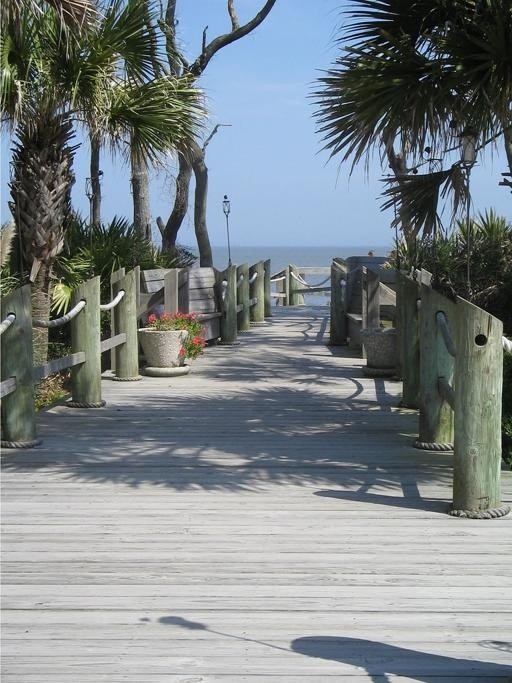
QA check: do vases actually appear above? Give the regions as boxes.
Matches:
[360,328,398,368]
[139,328,188,368]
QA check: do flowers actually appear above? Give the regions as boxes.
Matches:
[151,310,207,359]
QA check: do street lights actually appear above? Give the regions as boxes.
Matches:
[460,123,481,302]
[222,193,231,265]
